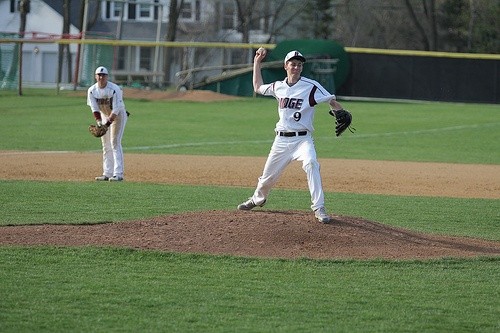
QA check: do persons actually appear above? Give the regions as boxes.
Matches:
[86,66,127,182]
[237,46,352,223]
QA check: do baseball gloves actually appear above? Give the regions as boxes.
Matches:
[88,123,109,138]
[329,109,357,137]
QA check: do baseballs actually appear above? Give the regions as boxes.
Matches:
[258,48,265,55]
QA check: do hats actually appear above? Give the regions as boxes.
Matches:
[95,65,108,74]
[285,50,306,65]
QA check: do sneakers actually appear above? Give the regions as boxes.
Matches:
[95,174,123,180]
[238,197,266,210]
[315,207,329,223]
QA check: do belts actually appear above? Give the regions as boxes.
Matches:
[277,130,307,137]
